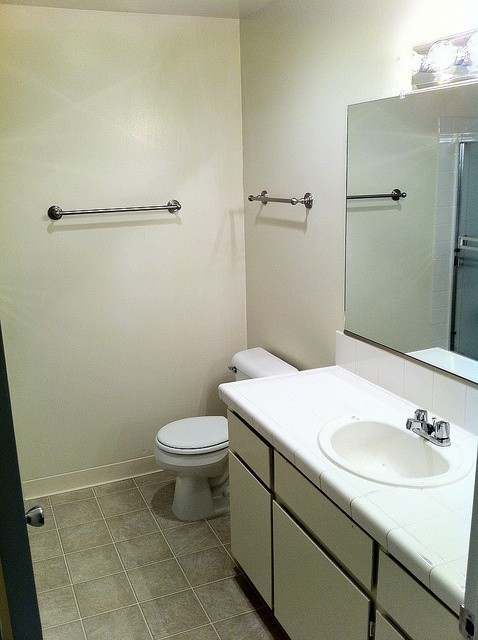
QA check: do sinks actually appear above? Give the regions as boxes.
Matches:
[218,331,478,628]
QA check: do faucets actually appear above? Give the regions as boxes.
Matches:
[406,410,428,430]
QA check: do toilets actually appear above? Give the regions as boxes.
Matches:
[153,347,300,521]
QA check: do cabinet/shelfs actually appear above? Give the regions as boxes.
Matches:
[374,548,464,639]
[229,411,272,618]
[272,450,377,639]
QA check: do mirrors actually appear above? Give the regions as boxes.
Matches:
[342,80,478,384]
